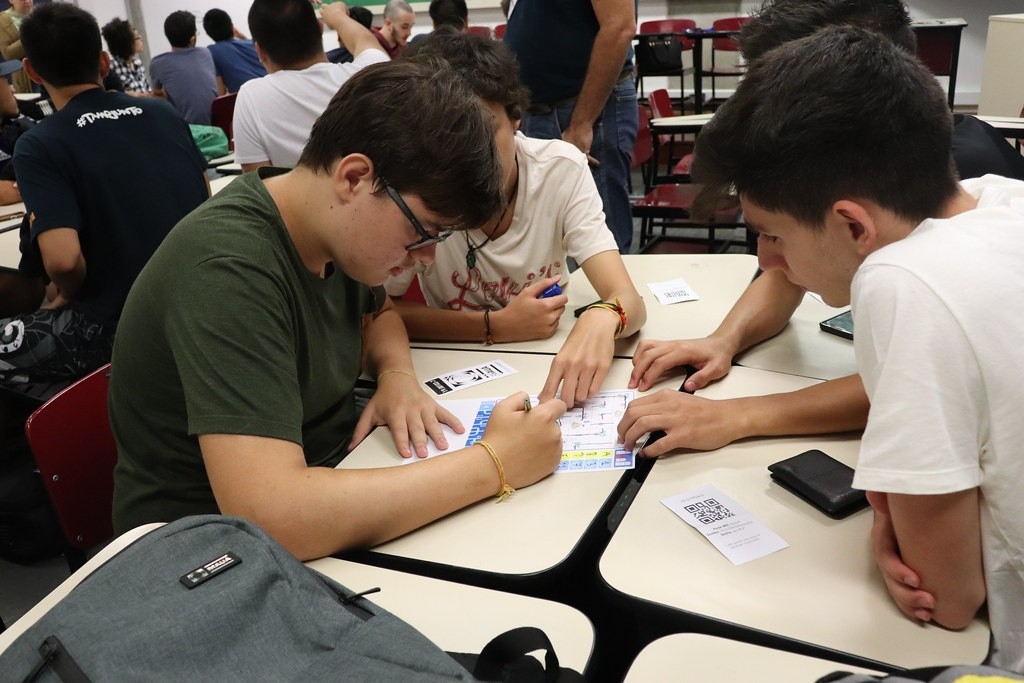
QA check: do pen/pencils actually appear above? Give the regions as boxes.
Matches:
[524,399,531,412]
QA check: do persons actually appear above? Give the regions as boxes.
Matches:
[0,0,212,384]
[148,11,219,125]
[112,54,567,561]
[203,8,268,95]
[338,7,373,48]
[501,0,639,255]
[384,26,647,408]
[0,59,23,206]
[616,0,1024,457]
[234,0,391,174]
[102,18,152,93]
[0,0,41,94]
[371,0,415,61]
[428,0,469,33]
[690,24,1024,676]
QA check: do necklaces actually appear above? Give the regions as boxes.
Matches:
[465,156,519,269]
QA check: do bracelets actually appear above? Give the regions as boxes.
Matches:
[483,310,493,345]
[377,370,414,382]
[474,441,515,503]
[587,298,627,340]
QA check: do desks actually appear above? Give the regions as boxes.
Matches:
[972,14,1024,153]
[0,255,990,683]
[649,113,715,136]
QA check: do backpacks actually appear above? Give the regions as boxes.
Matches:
[1,514,559,683]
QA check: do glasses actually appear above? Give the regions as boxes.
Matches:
[136,34,143,41]
[378,173,453,252]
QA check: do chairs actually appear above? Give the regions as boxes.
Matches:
[909,18,968,111]
[629,17,757,254]
[26,362,115,569]
[467,24,506,39]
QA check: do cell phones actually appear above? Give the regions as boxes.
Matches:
[818,309,853,340]
